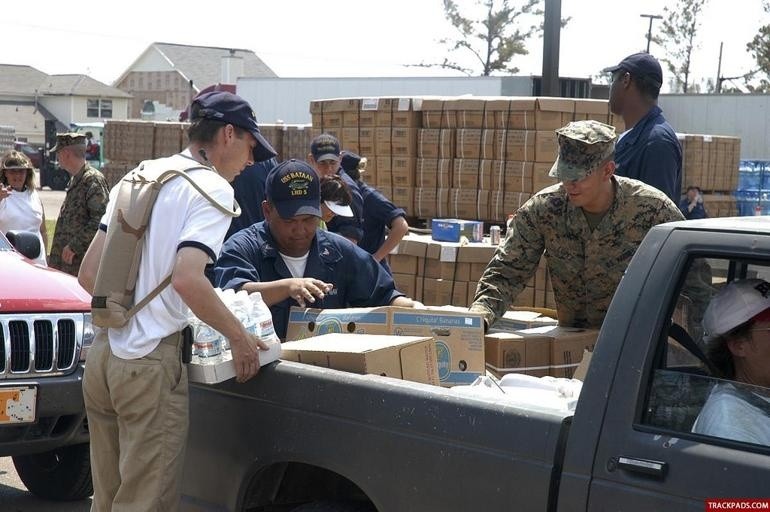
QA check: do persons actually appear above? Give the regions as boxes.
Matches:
[467,120,687,342]
[604,52,683,207]
[84,132,97,159]
[679,184,708,218]
[224,159,279,240]
[308,135,365,246]
[340,149,408,276]
[691,277,770,445]
[317,175,354,231]
[0,150,48,265]
[47,132,111,278]
[78,90,278,512]
[213,158,422,342]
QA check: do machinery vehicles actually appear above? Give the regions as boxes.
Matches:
[36,101,107,191]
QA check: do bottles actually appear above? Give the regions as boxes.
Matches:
[490,226,501,246]
[188,288,277,363]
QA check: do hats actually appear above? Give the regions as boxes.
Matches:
[548,120,618,182]
[3,158,33,170]
[702,277,770,344]
[49,132,87,153]
[310,131,341,164]
[603,51,663,88]
[265,157,323,219]
[190,91,279,163]
[319,178,355,218]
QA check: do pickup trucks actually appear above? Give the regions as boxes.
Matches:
[180,215,770,511]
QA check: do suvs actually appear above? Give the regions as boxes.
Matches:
[0,229,94,502]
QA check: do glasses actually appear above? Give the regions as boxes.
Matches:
[750,327,770,333]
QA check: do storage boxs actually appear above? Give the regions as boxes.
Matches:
[516,326,602,379]
[457,112,496,130]
[416,128,454,159]
[493,132,560,163]
[452,157,493,190]
[98,118,189,192]
[431,219,483,242]
[376,111,422,128]
[285,302,485,387]
[388,253,425,276]
[681,194,736,218]
[376,158,415,187]
[256,122,314,163]
[455,130,497,160]
[414,187,449,220]
[492,162,561,195]
[310,128,342,152]
[469,262,557,312]
[484,330,553,381]
[391,275,423,302]
[279,332,441,388]
[490,190,532,223]
[422,111,458,128]
[496,109,562,131]
[377,189,414,218]
[414,158,453,187]
[374,128,417,157]
[342,130,374,156]
[422,280,467,304]
[673,132,741,194]
[312,113,345,129]
[426,257,471,282]
[342,111,374,127]
[361,156,375,183]
[448,189,490,222]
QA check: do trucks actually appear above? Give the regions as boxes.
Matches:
[14,142,41,168]
[177,75,593,125]
